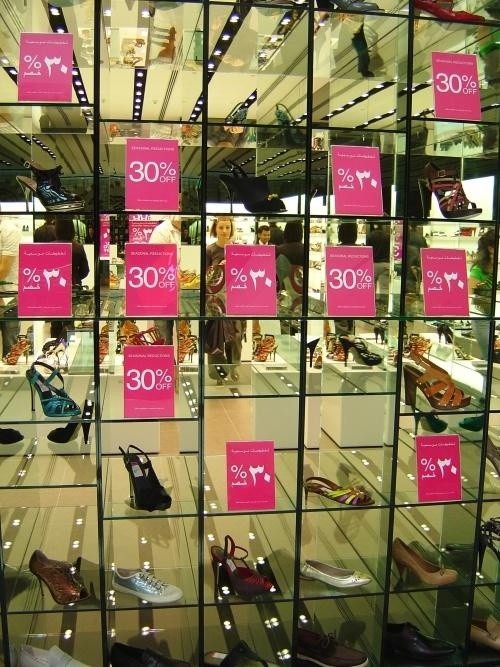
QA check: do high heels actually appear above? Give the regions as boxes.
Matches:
[1,321,385,365]
[274,101,306,146]
[210,534,276,597]
[16,156,86,212]
[217,158,288,215]
[277,254,303,302]
[316,0,385,13]
[117,444,172,512]
[28,548,90,606]
[313,132,325,151]
[404,323,485,436]
[416,160,483,218]
[392,537,459,586]
[412,0,484,22]
[26,361,82,418]
[478,517,499,571]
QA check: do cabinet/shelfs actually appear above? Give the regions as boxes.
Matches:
[4,1,497,664]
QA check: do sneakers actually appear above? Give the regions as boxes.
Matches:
[111,566,185,605]
[296,628,369,667]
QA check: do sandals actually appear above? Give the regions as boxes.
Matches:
[179,269,200,290]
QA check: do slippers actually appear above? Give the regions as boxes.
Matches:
[303,475,377,506]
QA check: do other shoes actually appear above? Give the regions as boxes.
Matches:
[220,640,268,667]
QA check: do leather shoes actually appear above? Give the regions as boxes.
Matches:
[110,643,191,667]
[385,621,456,660]
[18,644,91,667]
[298,559,372,590]
[469,614,500,651]
